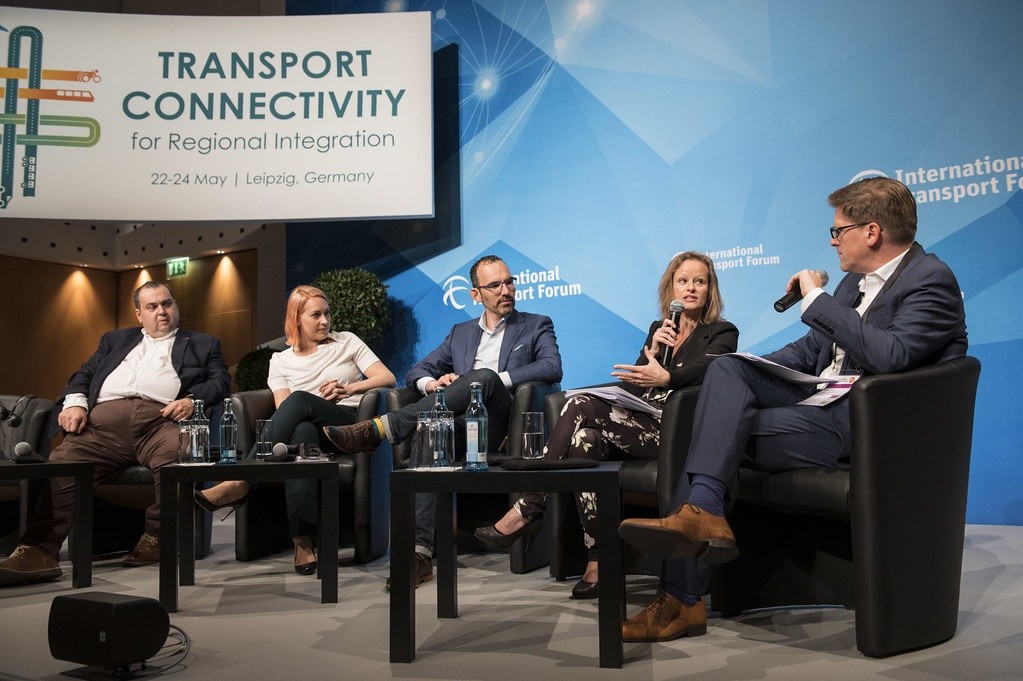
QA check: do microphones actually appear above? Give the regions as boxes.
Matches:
[773,269,829,313]
[14,442,45,462]
[272,443,298,457]
[663,299,685,367]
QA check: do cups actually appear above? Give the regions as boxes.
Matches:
[177,419,212,463]
[256,419,274,460]
[521,412,545,459]
[417,410,455,468]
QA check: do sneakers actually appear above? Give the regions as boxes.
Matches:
[0,543,63,578]
[323,415,383,455]
[122,532,159,565]
[386,551,434,592]
[622,587,708,642]
[617,502,738,564]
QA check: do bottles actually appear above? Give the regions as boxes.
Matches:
[219,398,238,464]
[431,386,448,411]
[465,382,488,471]
[192,399,206,419]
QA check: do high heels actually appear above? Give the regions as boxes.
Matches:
[572,579,598,599]
[473,516,542,553]
[294,542,317,575]
[195,487,254,522]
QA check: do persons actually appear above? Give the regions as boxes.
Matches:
[192,286,397,575]
[322,254,565,590]
[616,177,969,643]
[0,280,231,585]
[473,250,740,599]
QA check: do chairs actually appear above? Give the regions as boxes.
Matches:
[543,383,703,584]
[230,387,390,565]
[655,356,980,655]
[385,382,563,576]
[0,394,215,560]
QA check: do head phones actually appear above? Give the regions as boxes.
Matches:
[0,406,8,420]
[7,394,37,427]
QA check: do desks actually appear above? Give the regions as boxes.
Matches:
[159,459,339,611]
[0,460,95,586]
[385,461,622,671]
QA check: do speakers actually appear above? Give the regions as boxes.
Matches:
[47,591,170,669]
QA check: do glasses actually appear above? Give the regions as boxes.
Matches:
[829,221,883,239]
[477,277,517,293]
